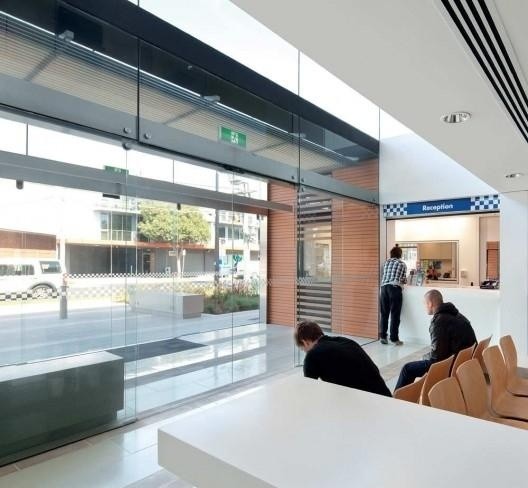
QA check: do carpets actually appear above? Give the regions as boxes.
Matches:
[105,338,209,362]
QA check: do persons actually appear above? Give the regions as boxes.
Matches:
[293,321,392,397]
[378,243,407,346]
[393,289,477,390]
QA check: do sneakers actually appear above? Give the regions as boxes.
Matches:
[391,341,403,345]
[380,338,388,344]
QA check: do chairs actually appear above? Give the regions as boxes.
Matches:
[392,332,528,431]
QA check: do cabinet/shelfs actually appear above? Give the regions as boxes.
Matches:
[418,243,452,260]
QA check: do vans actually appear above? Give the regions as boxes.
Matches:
[0,259,67,299]
